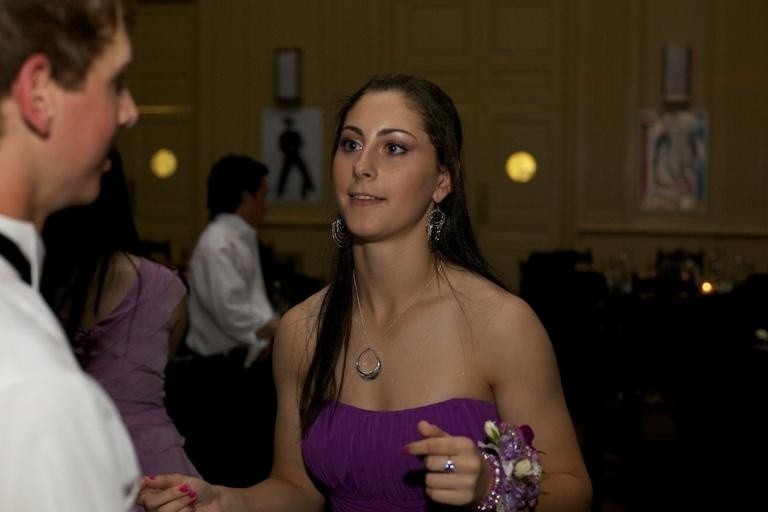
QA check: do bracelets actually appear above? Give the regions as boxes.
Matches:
[471,417,553,512]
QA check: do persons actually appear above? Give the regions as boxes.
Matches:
[40,141,206,512]
[271,116,316,201]
[0,1,146,512]
[182,153,282,487]
[135,72,596,512]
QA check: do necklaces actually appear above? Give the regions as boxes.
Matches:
[350,254,448,382]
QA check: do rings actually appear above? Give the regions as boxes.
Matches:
[444,454,457,474]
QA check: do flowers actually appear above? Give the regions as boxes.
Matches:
[479,416,546,512]
